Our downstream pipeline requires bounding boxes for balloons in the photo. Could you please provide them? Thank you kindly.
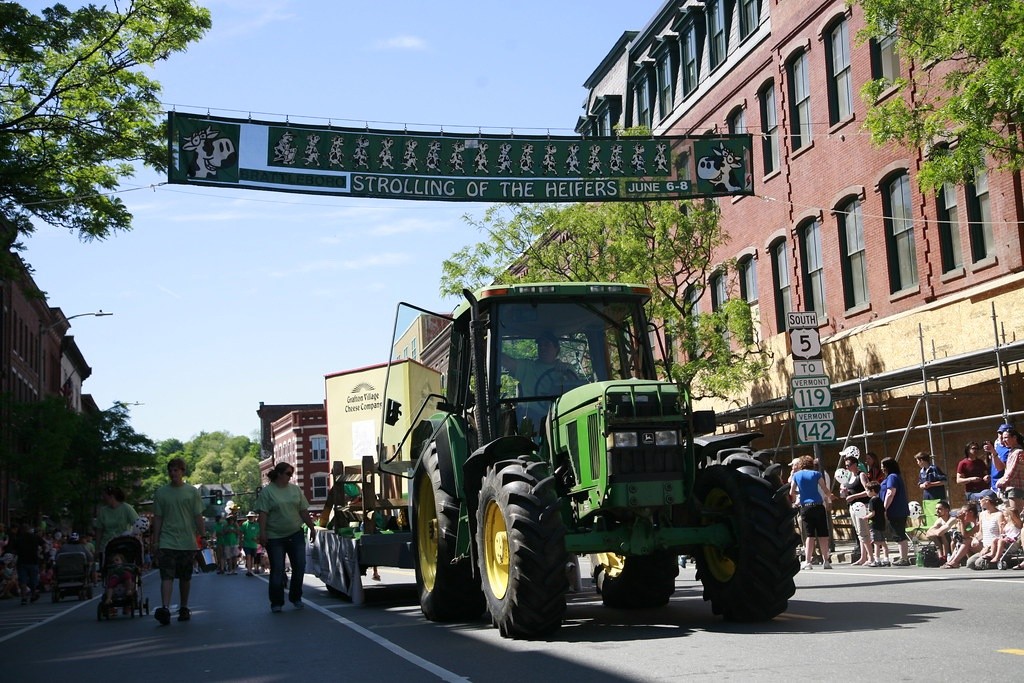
[843,446,860,461]
[850,502,866,518]
[835,468,848,484]
[907,502,922,518]
[134,516,149,533]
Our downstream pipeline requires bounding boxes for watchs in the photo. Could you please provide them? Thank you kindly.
[200,535,209,540]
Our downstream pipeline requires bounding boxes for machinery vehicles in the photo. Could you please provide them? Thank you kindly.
[301,282,803,641]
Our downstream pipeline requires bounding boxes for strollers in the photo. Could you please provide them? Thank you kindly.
[96,533,149,621]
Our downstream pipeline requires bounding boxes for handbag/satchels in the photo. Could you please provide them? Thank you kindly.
[916,546,939,567]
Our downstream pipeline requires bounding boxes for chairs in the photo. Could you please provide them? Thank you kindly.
[903,499,951,565]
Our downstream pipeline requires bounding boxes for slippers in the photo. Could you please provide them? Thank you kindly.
[940,562,960,568]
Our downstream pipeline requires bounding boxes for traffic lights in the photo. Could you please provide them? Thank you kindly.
[256,487,262,498]
[215,490,222,505]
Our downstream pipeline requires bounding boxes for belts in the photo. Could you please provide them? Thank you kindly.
[804,502,821,506]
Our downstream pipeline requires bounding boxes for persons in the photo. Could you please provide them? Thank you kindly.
[913,423,1024,570]
[348,485,397,581]
[0,521,96,610]
[839,446,910,567]
[787,456,835,570]
[193,510,270,575]
[485,332,587,419]
[94,486,150,621]
[258,463,317,613]
[302,511,322,537]
[154,459,210,625]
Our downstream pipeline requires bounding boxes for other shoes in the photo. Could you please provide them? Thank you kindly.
[127,589,133,596]
[986,553,995,558]
[853,557,909,567]
[802,563,813,570]
[823,563,832,569]
[193,564,264,576]
[158,610,171,625]
[271,602,282,612]
[104,599,113,604]
[990,557,1001,562]
[21,594,41,606]
[293,600,304,608]
[178,607,191,620]
[1012,564,1024,570]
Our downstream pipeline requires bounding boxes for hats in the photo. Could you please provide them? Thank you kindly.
[971,490,997,501]
[247,511,257,517]
[997,424,1012,432]
[788,458,801,466]
[68,532,80,541]
[225,515,235,520]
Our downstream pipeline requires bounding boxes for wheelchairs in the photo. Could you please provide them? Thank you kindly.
[49,551,93,603]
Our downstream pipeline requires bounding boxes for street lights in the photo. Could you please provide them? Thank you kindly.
[39,309,112,402]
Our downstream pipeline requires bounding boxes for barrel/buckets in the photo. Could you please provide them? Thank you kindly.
[195,548,219,574]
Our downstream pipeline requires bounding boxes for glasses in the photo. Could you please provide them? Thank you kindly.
[998,431,1003,435]
[979,497,987,500]
[283,471,292,476]
[844,462,856,468]
[970,447,979,451]
